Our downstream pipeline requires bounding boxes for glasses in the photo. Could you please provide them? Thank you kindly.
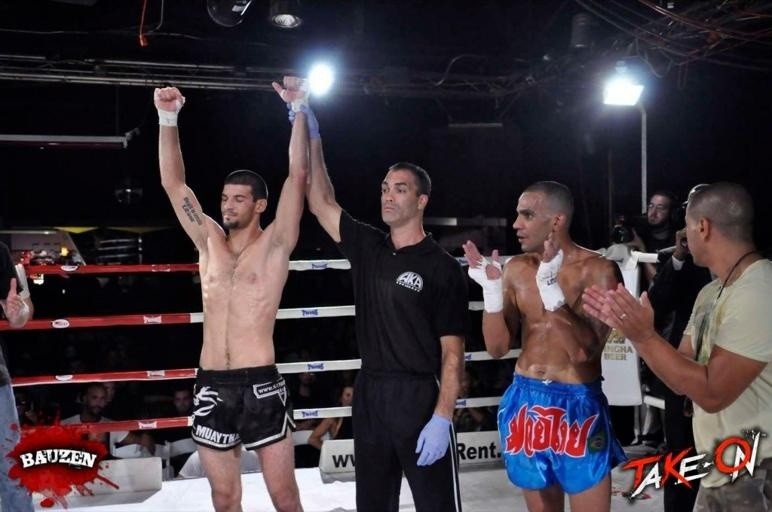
[647,203,671,211]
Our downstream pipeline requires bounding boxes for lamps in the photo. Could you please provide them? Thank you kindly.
[207,0,253,28]
[601,62,644,106]
[269,0,304,29]
[569,13,592,48]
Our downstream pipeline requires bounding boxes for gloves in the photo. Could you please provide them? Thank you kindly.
[287,104,321,140]
[415,414,451,466]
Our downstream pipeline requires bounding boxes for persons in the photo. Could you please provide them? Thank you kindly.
[460,181,628,512]
[152,76,310,512]
[287,102,468,512]
[0,242,35,512]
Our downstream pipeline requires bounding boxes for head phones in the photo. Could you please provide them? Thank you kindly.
[670,191,680,223]
